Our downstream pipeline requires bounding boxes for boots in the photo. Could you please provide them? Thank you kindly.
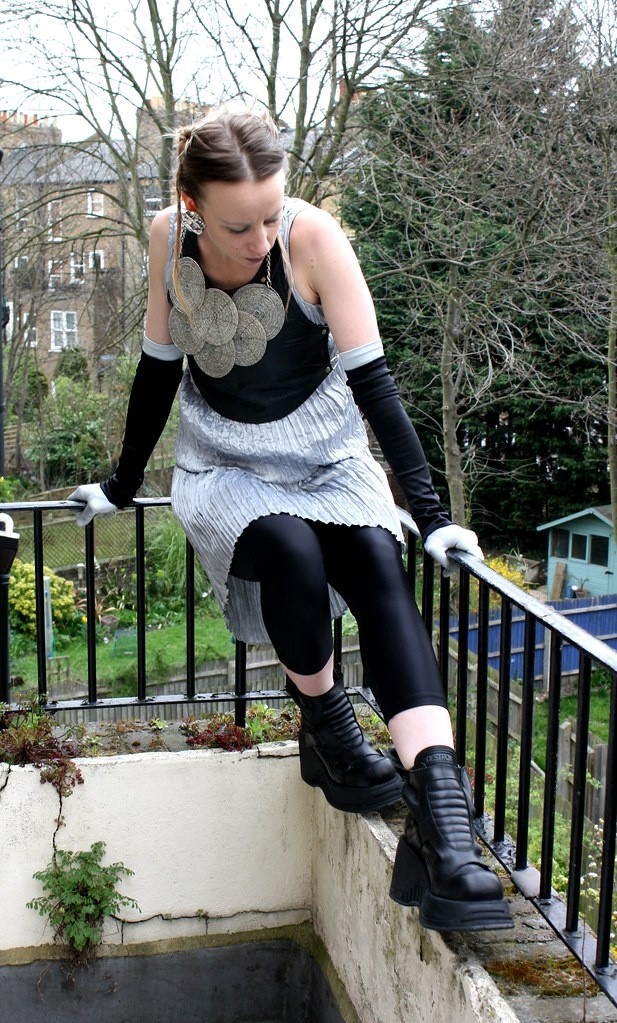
[286,668,405,813]
[388,744,515,932]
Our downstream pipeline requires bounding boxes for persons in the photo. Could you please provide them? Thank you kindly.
[70,107,514,933]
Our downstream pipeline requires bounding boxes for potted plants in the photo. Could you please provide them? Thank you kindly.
[568,574,590,598]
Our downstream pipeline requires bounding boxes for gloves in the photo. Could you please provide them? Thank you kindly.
[66,483,118,528]
[423,524,484,577]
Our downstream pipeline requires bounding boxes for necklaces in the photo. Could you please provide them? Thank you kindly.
[167,230,287,378]
